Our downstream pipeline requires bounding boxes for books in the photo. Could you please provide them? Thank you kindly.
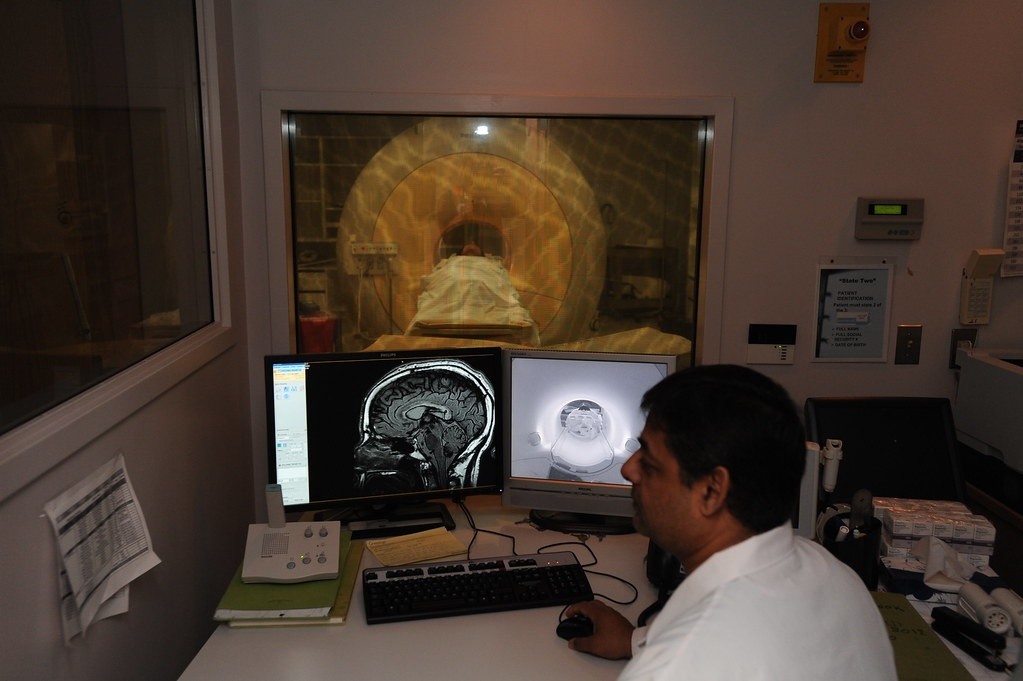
[212,530,365,628]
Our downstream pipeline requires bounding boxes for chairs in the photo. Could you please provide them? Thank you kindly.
[805,396,1001,599]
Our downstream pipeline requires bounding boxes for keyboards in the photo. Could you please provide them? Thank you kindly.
[363,550,594,624]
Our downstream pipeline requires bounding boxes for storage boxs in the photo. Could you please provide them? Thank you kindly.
[870,494,997,570]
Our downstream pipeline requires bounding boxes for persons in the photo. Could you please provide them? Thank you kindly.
[565,364,901,681]
[444,240,499,323]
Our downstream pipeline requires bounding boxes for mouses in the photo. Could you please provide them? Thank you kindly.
[556,616,595,642]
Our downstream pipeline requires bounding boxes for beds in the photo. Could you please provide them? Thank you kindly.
[410,253,535,345]
[550,428,614,473]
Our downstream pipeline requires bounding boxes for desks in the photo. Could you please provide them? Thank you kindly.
[165,504,659,681]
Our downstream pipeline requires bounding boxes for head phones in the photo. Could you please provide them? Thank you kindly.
[646,540,687,598]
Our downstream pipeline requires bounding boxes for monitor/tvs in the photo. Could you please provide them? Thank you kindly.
[505,348,677,535]
[264,347,505,539]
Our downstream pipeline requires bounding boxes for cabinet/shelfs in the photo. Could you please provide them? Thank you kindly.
[296,134,394,243]
[297,266,336,311]
